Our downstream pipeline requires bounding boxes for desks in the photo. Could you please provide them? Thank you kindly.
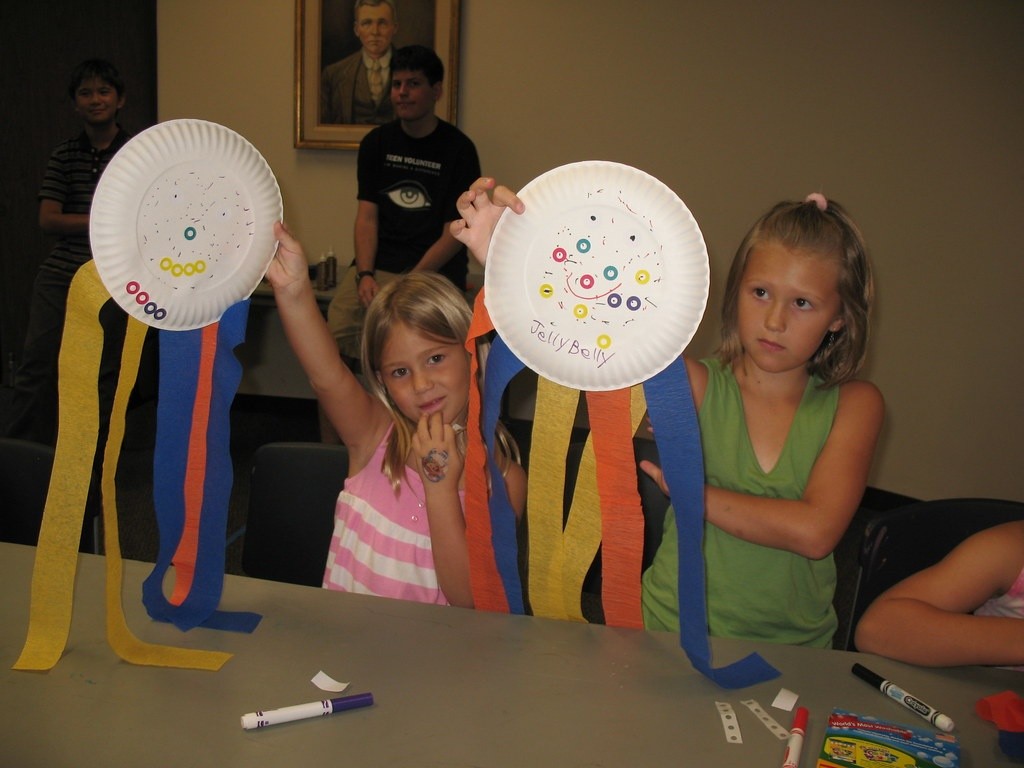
[250,259,484,318]
[0,541,1024,768]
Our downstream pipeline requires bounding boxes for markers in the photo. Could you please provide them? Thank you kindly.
[240,692,374,730]
[781,707,809,768]
[851,661,956,733]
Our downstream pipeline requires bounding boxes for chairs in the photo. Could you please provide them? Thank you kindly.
[845,496,1024,654]
[217,387,917,671]
[0,438,101,558]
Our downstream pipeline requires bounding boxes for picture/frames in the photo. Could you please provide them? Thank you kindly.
[293,0,462,151]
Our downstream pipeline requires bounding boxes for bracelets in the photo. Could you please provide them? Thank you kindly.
[353,269,376,284]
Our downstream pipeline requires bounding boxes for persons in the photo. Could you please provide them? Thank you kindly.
[266,220,527,611]
[854,518,1024,668]
[450,175,885,648]
[2,56,133,443]
[314,44,481,447]
